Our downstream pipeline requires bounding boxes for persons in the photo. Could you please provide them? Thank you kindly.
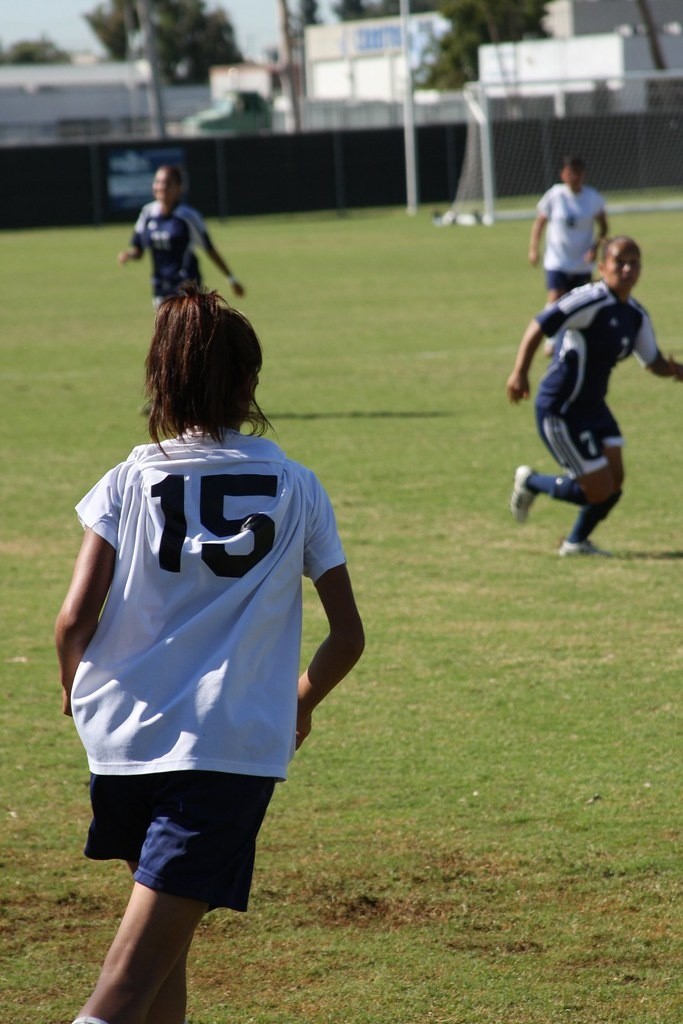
[119,163,244,314]
[508,236,683,560]
[53,284,364,1024]
[528,156,609,356]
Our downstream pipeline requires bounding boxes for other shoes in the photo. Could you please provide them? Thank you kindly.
[544,334,558,357]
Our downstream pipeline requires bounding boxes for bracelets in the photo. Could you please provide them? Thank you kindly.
[228,275,239,284]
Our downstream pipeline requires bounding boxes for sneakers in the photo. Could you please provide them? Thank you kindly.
[559,538,612,559]
[509,465,537,524]
